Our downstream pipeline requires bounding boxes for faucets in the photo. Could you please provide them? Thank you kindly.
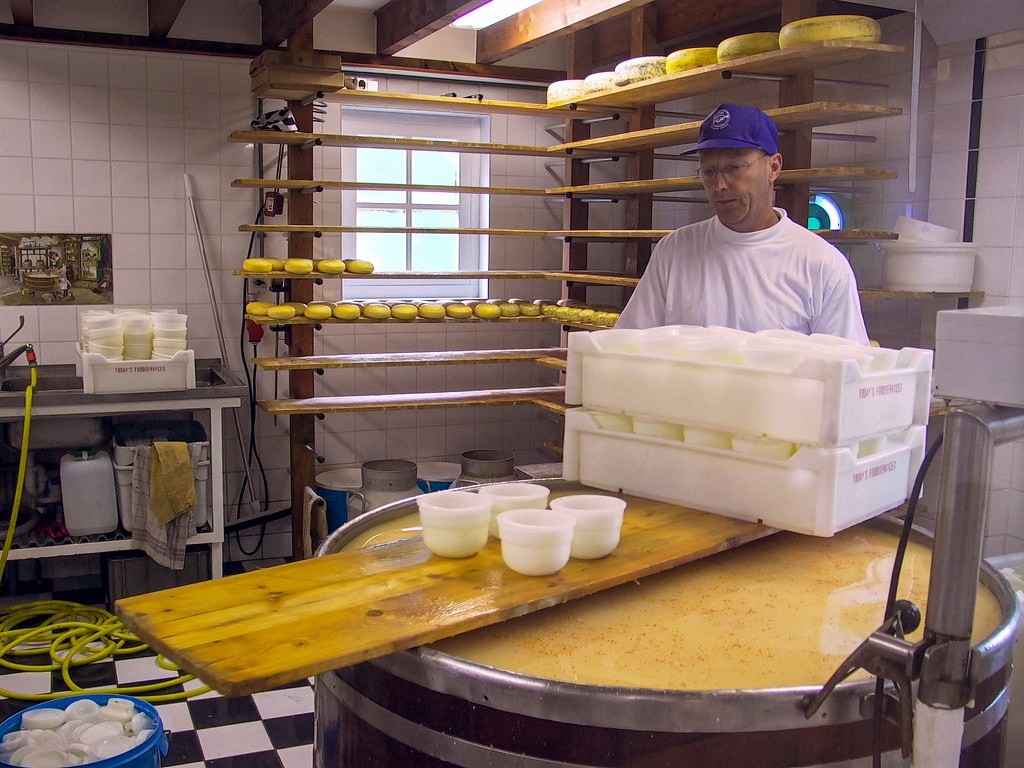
[0,314,39,381]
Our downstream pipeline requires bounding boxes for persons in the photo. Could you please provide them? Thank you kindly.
[612,103,871,348]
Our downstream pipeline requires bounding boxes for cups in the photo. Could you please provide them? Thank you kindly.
[549,495,628,560]
[496,508,578,576]
[416,491,492,558]
[478,483,551,540]
[80,308,189,362]
[589,412,900,463]
[591,323,900,378]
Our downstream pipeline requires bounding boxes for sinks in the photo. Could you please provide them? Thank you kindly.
[0,377,104,451]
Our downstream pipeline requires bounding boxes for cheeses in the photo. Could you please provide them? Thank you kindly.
[242,257,374,273]
[778,15,881,50]
[666,47,718,75]
[547,79,584,103]
[717,32,779,63]
[614,56,666,86]
[584,72,614,95]
[246,298,624,325]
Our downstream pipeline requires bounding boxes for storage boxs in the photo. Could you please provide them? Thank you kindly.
[251,68,345,101]
[75,340,196,395]
[933,305,1024,407]
[109,419,211,532]
[249,50,341,76]
[561,325,935,538]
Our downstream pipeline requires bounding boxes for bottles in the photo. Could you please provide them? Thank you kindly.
[59,449,119,537]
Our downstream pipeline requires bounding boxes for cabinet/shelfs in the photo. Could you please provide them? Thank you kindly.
[1,397,241,580]
[227,36,915,417]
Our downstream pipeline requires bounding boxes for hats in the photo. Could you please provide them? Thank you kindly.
[679,103,778,156]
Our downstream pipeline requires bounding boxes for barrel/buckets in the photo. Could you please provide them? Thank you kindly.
[315,449,518,534]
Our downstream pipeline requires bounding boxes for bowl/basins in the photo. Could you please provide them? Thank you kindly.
[876,240,979,293]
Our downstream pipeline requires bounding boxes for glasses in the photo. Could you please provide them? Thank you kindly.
[694,153,767,179]
